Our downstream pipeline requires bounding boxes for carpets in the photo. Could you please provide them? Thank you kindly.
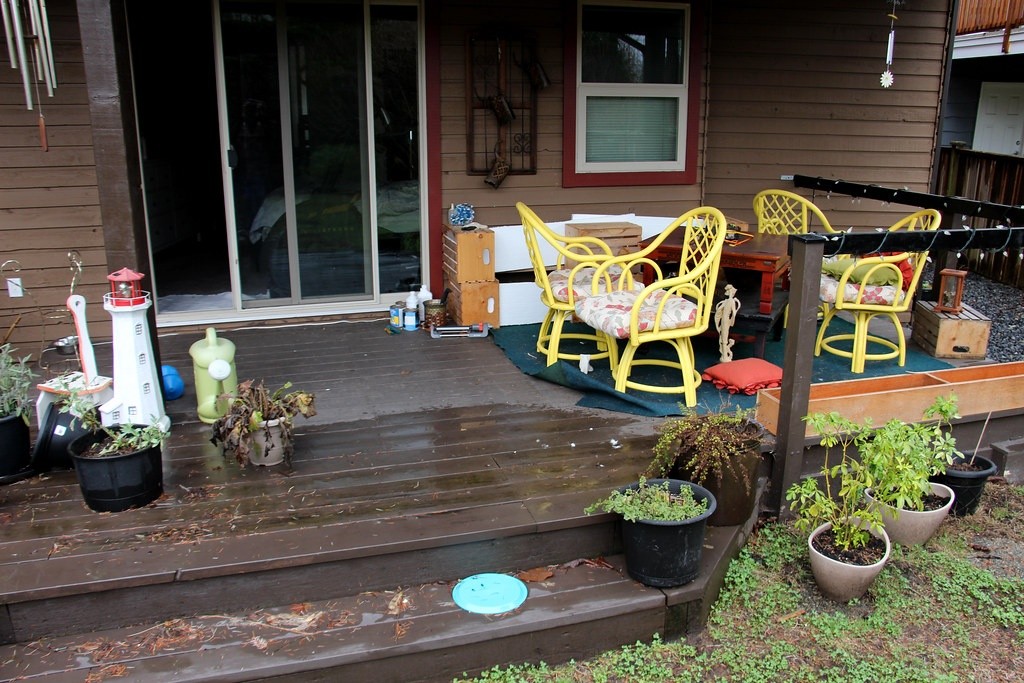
[491,316,957,417]
[158,289,270,314]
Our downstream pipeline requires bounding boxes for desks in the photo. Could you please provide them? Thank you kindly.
[638,225,790,360]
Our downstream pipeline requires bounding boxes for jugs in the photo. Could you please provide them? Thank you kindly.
[189,327,239,425]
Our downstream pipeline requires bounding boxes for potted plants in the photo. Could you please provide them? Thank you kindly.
[0,343,41,475]
[786,390,996,600]
[583,392,779,587]
[209,377,318,469]
[54,371,171,512]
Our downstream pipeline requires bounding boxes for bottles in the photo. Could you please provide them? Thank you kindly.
[417,286,432,321]
[161,364,185,400]
[406,290,419,310]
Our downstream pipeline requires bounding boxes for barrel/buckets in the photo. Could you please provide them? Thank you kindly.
[389,301,421,331]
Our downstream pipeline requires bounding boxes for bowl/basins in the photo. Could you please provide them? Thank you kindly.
[53,336,79,355]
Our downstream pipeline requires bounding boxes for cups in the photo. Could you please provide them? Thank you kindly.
[422,299,448,327]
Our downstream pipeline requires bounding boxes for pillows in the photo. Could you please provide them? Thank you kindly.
[821,258,899,287]
[702,357,783,395]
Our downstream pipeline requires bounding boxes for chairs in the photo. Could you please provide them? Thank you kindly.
[753,189,941,373]
[515,202,727,409]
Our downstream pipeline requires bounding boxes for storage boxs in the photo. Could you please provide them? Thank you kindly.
[755,363,1024,437]
[566,223,644,323]
[932,267,968,315]
[911,300,992,361]
[442,223,501,330]
